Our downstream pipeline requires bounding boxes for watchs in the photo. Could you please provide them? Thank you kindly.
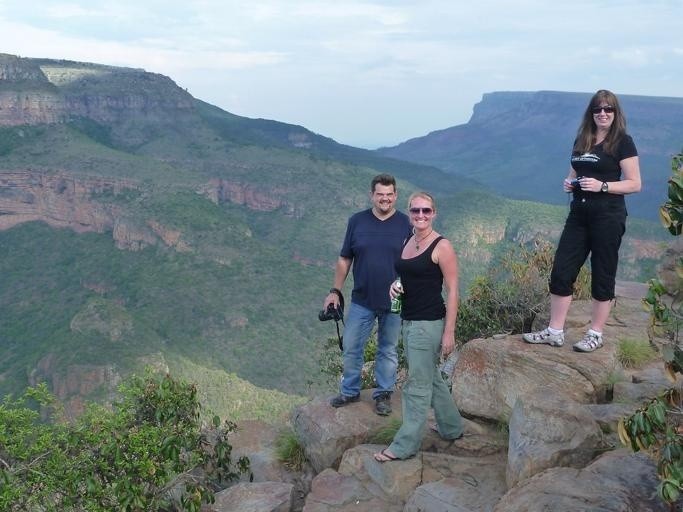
[601,179,609,195]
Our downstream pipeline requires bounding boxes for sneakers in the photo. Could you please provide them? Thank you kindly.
[375,393,392,415]
[573,332,603,352]
[330,393,360,406]
[523,329,564,346]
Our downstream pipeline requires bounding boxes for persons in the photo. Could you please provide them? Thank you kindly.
[373,190,458,464]
[323,172,411,415]
[521,89,643,352]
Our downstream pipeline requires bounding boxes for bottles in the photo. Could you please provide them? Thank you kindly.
[390,277,402,315]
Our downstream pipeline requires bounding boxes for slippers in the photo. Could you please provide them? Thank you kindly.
[375,448,402,463]
[431,424,464,438]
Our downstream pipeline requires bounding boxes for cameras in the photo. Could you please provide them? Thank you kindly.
[571,180,581,193]
[319,304,345,321]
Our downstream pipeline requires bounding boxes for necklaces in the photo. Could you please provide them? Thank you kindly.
[413,229,434,252]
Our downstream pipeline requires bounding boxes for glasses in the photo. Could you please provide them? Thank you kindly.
[591,105,616,113]
[410,208,432,216]
